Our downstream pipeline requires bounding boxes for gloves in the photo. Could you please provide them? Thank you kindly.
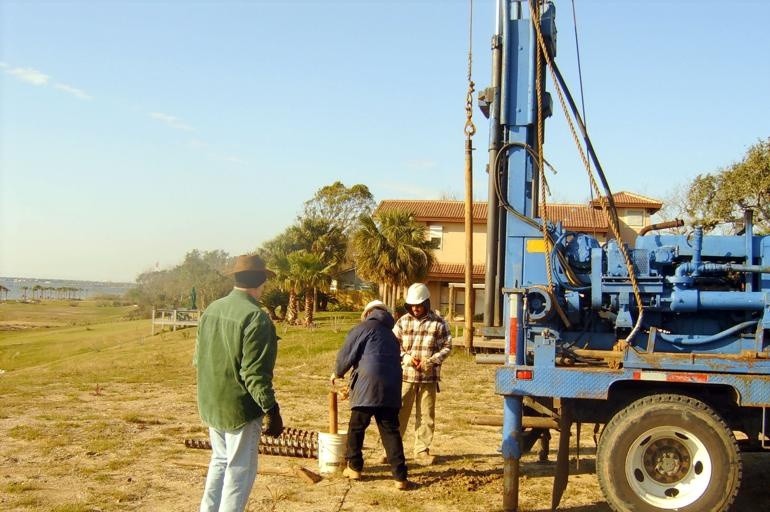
[261,404,283,438]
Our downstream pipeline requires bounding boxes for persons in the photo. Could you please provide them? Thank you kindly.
[392,282,452,466]
[196,254,284,512]
[330,300,409,490]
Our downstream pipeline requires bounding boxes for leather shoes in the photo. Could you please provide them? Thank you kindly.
[413,452,436,462]
[394,479,408,489]
[343,468,361,479]
[378,455,387,464]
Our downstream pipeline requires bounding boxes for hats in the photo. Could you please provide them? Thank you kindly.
[224,254,276,278]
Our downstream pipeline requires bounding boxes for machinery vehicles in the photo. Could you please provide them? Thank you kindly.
[460,1,769,512]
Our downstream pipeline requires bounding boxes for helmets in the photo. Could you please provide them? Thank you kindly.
[360,299,387,321]
[405,282,431,305]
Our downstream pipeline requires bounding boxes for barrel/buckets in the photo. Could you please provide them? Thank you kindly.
[318,429,349,478]
